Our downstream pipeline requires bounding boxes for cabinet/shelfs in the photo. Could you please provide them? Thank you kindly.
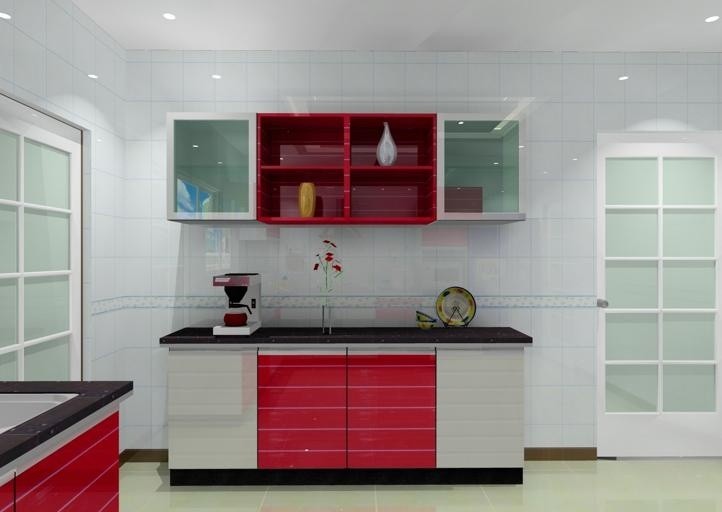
[256,114,438,223]
[437,115,526,221]
[168,349,258,470]
[437,351,524,471]
[1,410,121,512]
[166,114,257,221]
[257,347,435,470]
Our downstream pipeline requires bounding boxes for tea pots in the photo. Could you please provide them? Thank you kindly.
[223,304,252,327]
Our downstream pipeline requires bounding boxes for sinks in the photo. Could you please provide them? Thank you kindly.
[0,388,84,433]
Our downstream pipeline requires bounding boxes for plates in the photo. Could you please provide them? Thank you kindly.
[436,286,476,326]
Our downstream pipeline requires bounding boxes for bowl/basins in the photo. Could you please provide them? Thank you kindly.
[415,311,437,331]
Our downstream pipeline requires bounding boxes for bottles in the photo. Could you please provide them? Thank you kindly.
[298,182,316,218]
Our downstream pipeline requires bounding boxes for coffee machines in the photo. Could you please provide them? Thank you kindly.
[212,272,263,335]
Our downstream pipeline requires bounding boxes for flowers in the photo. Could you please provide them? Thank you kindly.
[312,239,346,299]
